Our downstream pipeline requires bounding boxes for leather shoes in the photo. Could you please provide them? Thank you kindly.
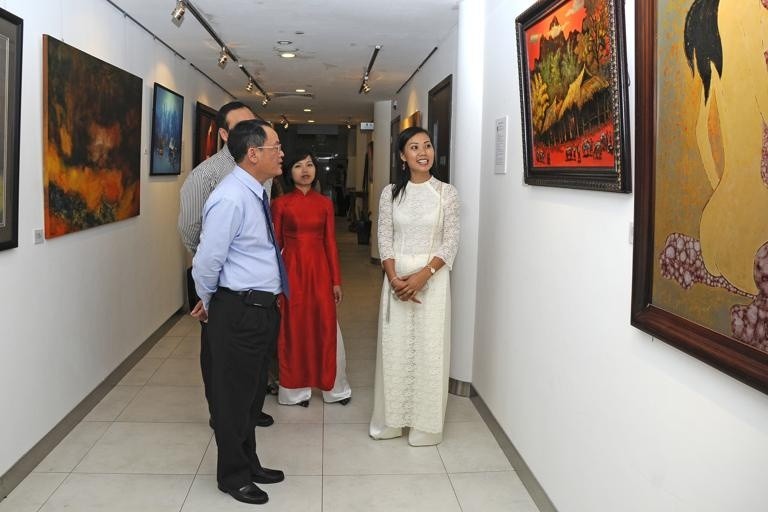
[256,413,274,426]
[218,482,269,504]
[255,467,285,484]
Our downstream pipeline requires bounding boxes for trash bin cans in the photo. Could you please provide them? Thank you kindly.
[355,221,371,244]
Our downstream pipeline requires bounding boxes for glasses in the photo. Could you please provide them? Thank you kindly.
[258,143,282,152]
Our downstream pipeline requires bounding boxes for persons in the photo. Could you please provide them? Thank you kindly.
[368,125,461,447]
[269,149,353,409]
[190,119,291,504]
[681,2,768,295]
[178,100,274,430]
[333,163,348,217]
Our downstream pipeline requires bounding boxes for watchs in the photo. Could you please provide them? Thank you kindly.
[425,263,436,275]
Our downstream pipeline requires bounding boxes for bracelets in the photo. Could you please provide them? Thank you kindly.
[389,276,399,287]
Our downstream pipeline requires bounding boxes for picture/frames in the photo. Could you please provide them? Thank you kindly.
[192,102,219,171]
[515,0,632,194]
[389,115,401,184]
[149,83,184,176]
[630,1,767,396]
[0,8,23,251]
[427,73,451,185]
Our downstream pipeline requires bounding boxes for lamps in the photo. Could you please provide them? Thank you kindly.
[171,1,269,109]
[277,113,291,129]
[361,72,371,96]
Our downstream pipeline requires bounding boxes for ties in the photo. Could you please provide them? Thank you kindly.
[263,191,289,299]
